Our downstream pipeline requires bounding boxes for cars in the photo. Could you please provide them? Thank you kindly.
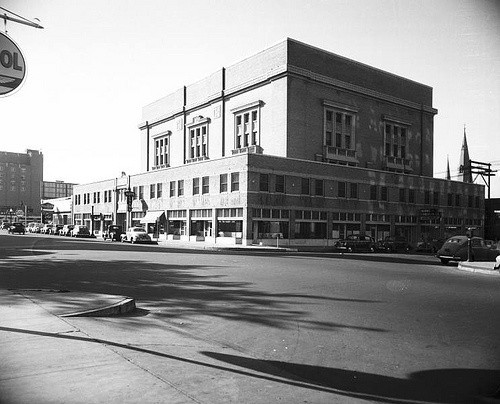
[437,236,500,265]
[2,221,151,243]
[338,234,378,253]
[377,234,416,253]
